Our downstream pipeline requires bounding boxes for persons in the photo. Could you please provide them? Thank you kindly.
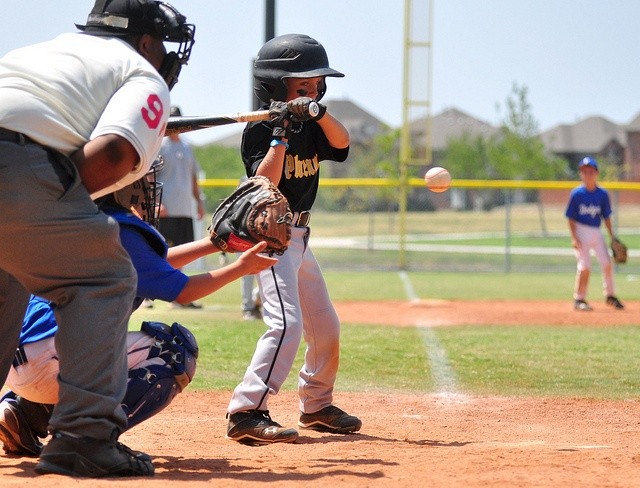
[566,157,627,313]
[142,105,206,308]
[0,0,195,477]
[224,34,361,443]
[0,152,278,460]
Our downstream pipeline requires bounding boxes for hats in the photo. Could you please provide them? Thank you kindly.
[579,158,597,168]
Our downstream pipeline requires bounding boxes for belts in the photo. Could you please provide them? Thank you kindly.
[0,127,39,145]
[290,211,311,227]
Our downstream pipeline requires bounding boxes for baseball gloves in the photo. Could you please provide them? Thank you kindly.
[210,172,294,256]
[611,238,629,264]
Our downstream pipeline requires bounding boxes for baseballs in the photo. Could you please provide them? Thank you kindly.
[426,166,452,192]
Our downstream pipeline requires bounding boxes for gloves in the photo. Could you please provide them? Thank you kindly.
[286,97,327,121]
[269,102,289,140]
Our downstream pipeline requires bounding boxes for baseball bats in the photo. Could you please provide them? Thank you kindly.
[162,100,320,136]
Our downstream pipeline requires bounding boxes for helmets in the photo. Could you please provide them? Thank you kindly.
[96,153,163,227]
[74,0,195,90]
[253,35,344,105]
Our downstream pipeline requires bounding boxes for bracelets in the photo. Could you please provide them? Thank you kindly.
[270,138,290,147]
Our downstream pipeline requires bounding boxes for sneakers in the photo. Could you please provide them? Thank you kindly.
[242,306,263,322]
[0,398,45,454]
[575,301,593,311]
[35,432,153,477]
[226,409,299,447]
[606,297,624,310]
[299,405,362,432]
